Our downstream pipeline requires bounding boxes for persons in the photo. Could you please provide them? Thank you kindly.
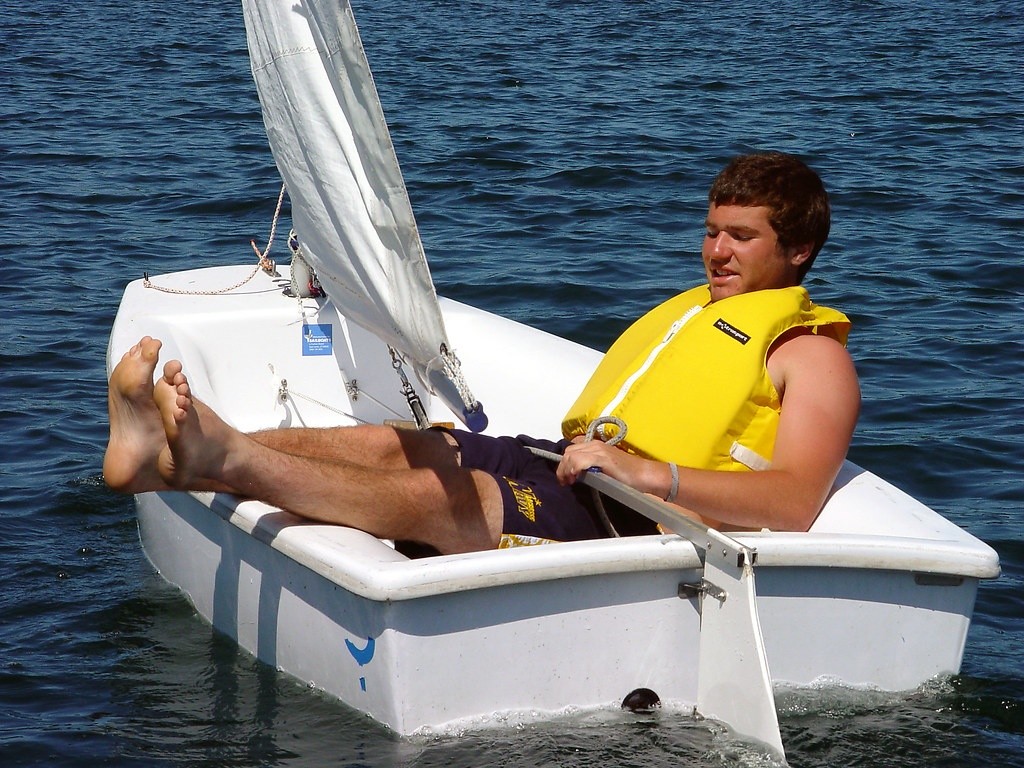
[101,154,861,560]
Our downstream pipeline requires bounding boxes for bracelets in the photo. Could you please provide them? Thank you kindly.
[663,463,679,504]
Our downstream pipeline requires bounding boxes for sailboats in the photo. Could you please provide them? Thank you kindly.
[101,1,1001,739]
